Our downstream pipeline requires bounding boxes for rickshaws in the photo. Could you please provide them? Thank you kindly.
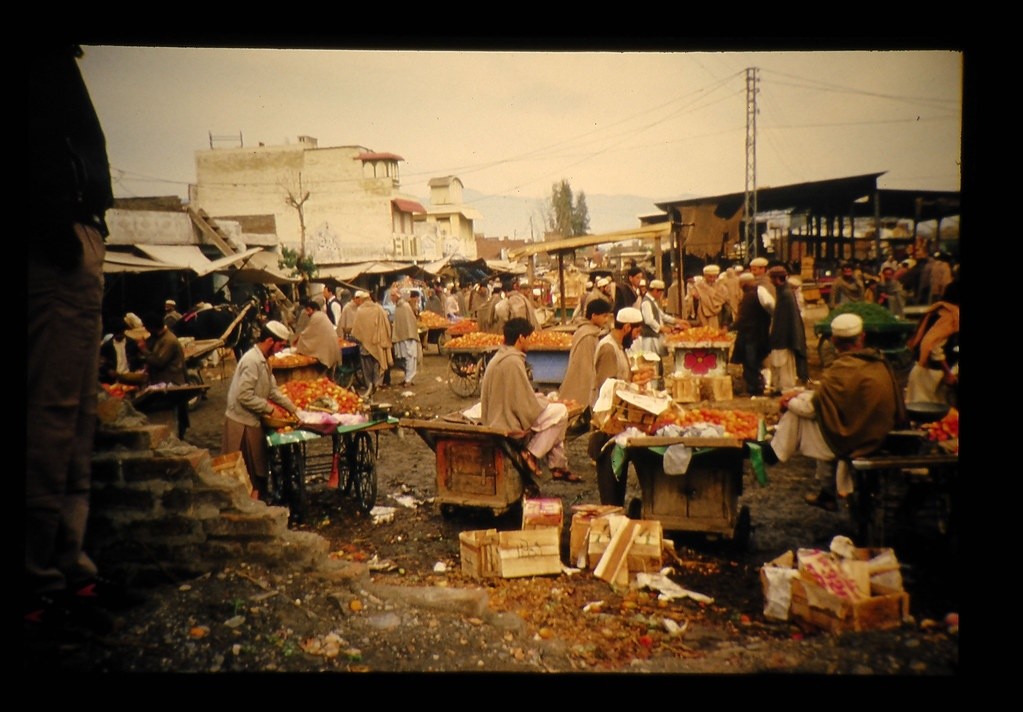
[123,345,958,549]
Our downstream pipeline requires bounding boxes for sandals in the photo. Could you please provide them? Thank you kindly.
[519,449,543,480]
[551,467,586,483]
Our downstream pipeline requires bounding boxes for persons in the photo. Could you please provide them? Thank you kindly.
[91,258,961,511]
[10,45,111,591]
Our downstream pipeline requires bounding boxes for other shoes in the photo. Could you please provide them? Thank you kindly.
[804,488,838,511]
[745,440,778,466]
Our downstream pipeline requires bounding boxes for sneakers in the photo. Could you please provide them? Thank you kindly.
[22,589,126,635]
[61,568,150,611]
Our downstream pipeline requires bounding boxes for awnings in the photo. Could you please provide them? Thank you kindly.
[101,246,528,291]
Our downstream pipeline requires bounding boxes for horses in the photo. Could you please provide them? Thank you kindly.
[170,282,277,362]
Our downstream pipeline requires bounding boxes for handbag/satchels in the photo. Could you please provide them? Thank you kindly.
[588,431,610,462]
[756,284,775,315]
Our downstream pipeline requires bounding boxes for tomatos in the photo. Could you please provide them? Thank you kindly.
[276,376,364,433]
[103,382,134,399]
[675,408,758,440]
[925,420,959,442]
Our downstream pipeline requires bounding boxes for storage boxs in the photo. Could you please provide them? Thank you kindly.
[761,544,911,646]
[206,451,254,494]
[458,496,664,583]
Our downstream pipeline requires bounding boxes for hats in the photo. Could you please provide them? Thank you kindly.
[649,280,666,289]
[616,307,643,323]
[831,313,863,337]
[265,320,290,340]
[165,300,176,306]
[750,256,768,268]
[703,264,720,275]
[354,289,370,299]
[735,265,743,272]
[585,274,612,289]
[770,266,787,277]
